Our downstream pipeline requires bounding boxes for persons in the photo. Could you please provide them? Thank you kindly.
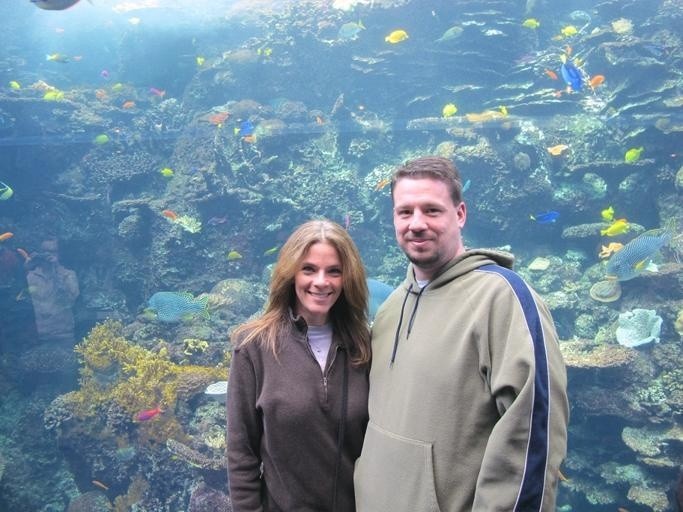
[351,155,571,512]
[224,218,372,512]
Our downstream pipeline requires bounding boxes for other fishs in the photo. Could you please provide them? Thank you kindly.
[434,0,683,283]
[1,0,279,263]
